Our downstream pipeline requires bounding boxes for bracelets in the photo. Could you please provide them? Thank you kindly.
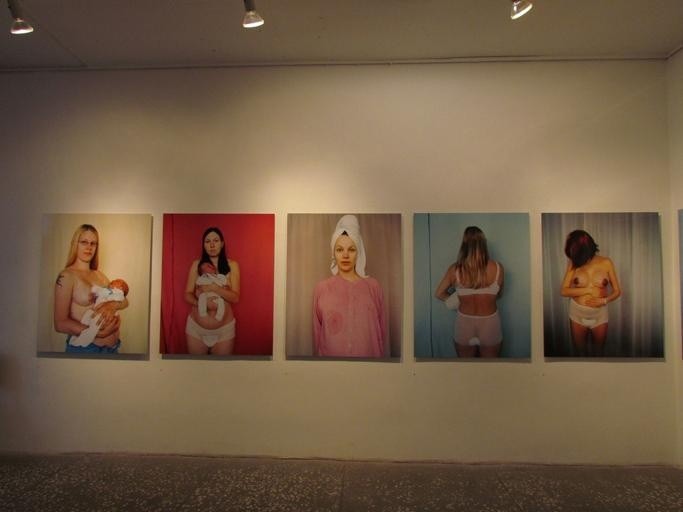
[605,299,608,306]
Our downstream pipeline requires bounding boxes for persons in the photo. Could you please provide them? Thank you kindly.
[183,227,241,356]
[435,226,504,358]
[53,224,129,354]
[197,260,231,323]
[311,214,386,357]
[81,279,128,338]
[559,229,621,356]
